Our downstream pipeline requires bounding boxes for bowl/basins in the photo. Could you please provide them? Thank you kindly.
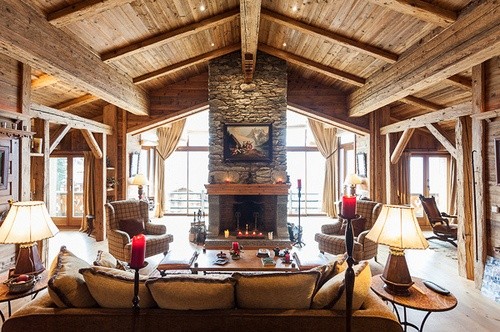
[7,274,35,293]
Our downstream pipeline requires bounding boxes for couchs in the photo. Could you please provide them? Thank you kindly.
[0,290,404,332]
[105,199,173,265]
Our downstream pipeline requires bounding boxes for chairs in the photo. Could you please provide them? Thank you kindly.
[419,194,458,247]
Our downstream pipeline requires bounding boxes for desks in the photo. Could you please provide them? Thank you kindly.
[371,274,457,332]
[189,249,299,276]
[0,267,51,323]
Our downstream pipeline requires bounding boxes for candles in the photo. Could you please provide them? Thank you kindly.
[342,193,359,218]
[298,179,302,188]
[132,234,145,266]
[232,242,240,253]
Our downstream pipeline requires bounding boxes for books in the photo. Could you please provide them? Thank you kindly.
[257,248,270,258]
[213,257,228,265]
[261,257,276,266]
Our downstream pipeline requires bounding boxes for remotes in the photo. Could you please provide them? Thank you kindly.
[422,279,450,295]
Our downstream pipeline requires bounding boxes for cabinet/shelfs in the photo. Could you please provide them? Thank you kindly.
[105,167,117,203]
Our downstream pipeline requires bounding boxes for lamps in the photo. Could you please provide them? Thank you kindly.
[365,203,428,294]
[346,174,363,198]
[132,174,148,201]
[0,201,59,276]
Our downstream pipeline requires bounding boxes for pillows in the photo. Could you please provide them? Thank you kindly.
[117,218,146,239]
[47,243,373,309]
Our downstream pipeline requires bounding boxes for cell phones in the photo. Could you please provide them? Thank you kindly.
[214,258,228,265]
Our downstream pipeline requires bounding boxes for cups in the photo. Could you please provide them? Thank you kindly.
[268,231,273,240]
[224,230,229,238]
[33,138,43,153]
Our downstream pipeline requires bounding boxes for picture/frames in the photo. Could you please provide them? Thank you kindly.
[223,123,273,164]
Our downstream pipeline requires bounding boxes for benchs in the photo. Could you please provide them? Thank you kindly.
[293,250,325,272]
[157,247,196,275]
